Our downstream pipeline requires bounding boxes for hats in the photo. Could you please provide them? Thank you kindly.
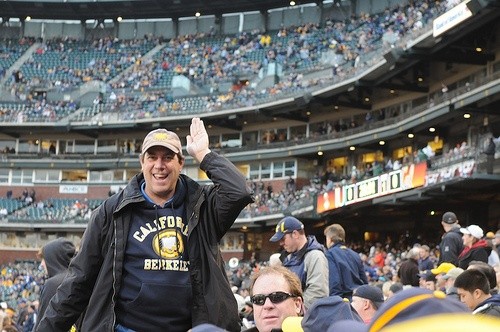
[460,225,484,239]
[353,285,383,309]
[140,129,182,157]
[430,263,456,274]
[442,212,457,224]
[443,268,463,279]
[269,216,304,242]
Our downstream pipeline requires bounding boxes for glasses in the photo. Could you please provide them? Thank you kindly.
[251,291,298,305]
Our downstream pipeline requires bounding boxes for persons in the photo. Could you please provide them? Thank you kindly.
[35,116,256,332]
[1,0,500,332]
